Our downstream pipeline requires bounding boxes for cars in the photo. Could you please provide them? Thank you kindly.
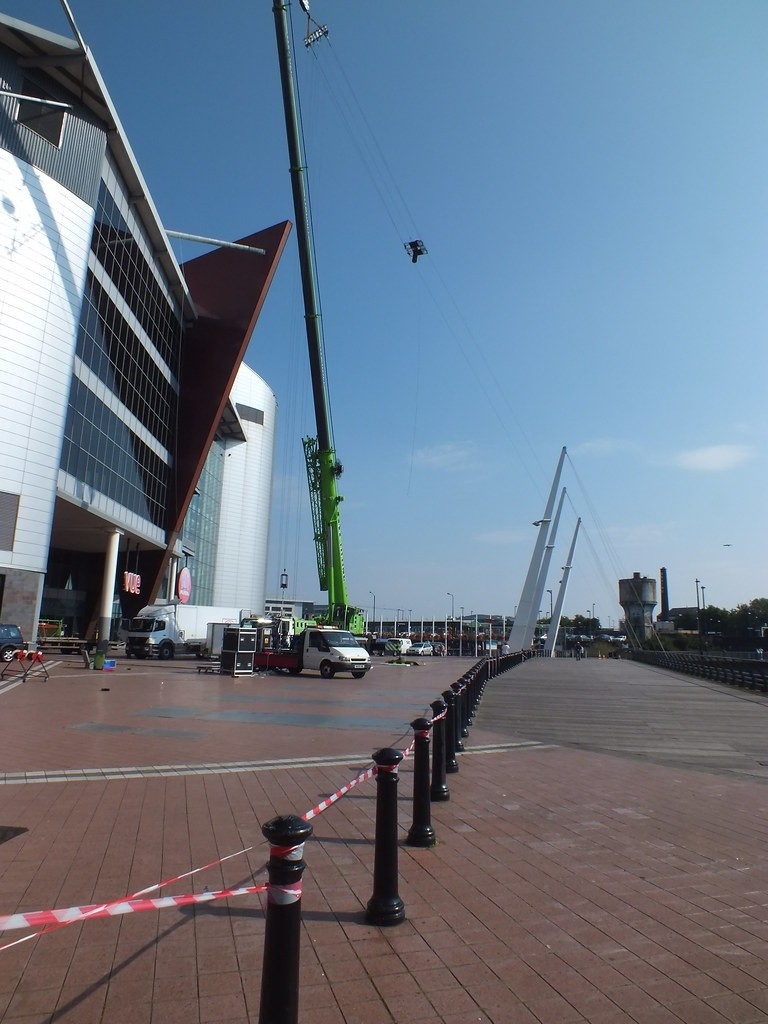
[344,637,446,657]
[531,632,627,645]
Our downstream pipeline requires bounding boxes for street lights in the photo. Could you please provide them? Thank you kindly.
[695,578,701,633]
[368,590,597,657]
[700,585,706,610]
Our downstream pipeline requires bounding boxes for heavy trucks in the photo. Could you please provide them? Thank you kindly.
[125,602,261,661]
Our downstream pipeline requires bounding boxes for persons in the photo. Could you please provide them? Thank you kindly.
[477,644,481,657]
[755,647,764,660]
[440,643,445,656]
[575,642,582,661]
[502,642,511,655]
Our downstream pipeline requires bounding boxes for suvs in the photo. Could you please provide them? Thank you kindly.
[0,623,24,662]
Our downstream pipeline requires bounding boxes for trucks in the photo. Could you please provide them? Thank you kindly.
[256,623,373,678]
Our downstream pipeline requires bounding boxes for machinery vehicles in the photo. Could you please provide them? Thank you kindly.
[267,0,379,655]
